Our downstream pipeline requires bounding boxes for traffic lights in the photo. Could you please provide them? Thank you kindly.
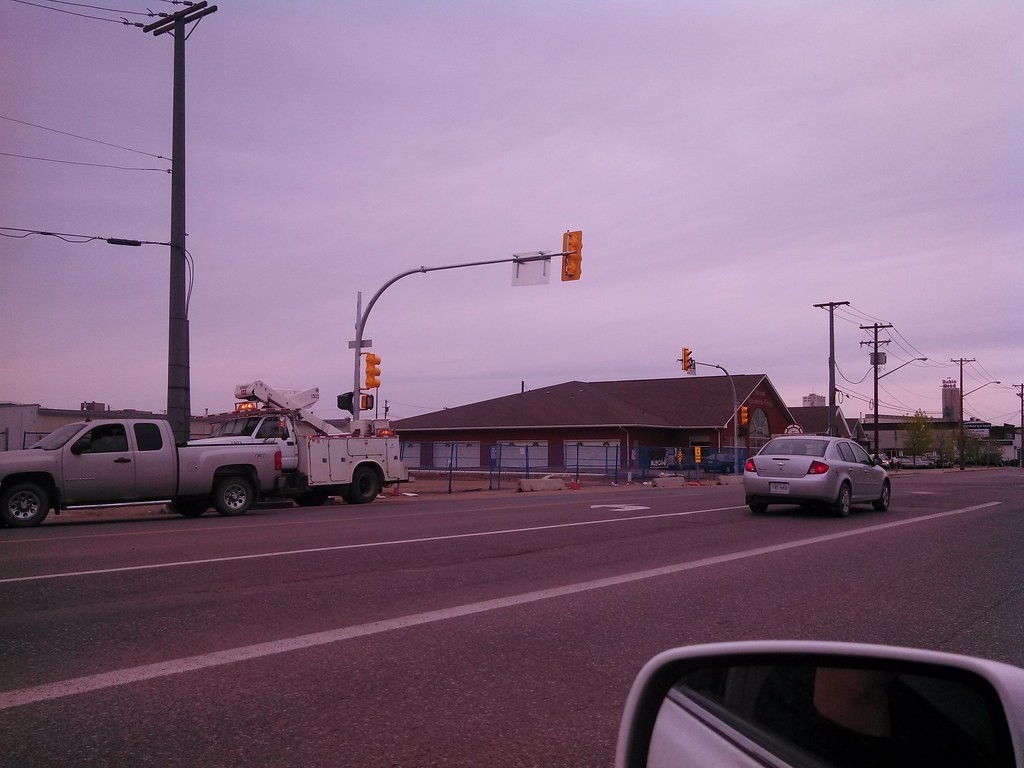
[561,230,582,280]
[741,406,749,426]
[682,347,692,370]
[366,353,381,387]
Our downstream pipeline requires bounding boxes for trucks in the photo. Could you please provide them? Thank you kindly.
[187,378,410,504]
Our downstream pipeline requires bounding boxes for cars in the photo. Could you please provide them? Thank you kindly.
[702,453,745,474]
[869,451,953,469]
[742,435,892,519]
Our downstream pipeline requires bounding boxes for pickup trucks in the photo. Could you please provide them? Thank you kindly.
[0,419,282,530]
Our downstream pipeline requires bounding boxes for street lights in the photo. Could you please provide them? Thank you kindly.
[859,322,927,461]
[951,358,1001,470]
